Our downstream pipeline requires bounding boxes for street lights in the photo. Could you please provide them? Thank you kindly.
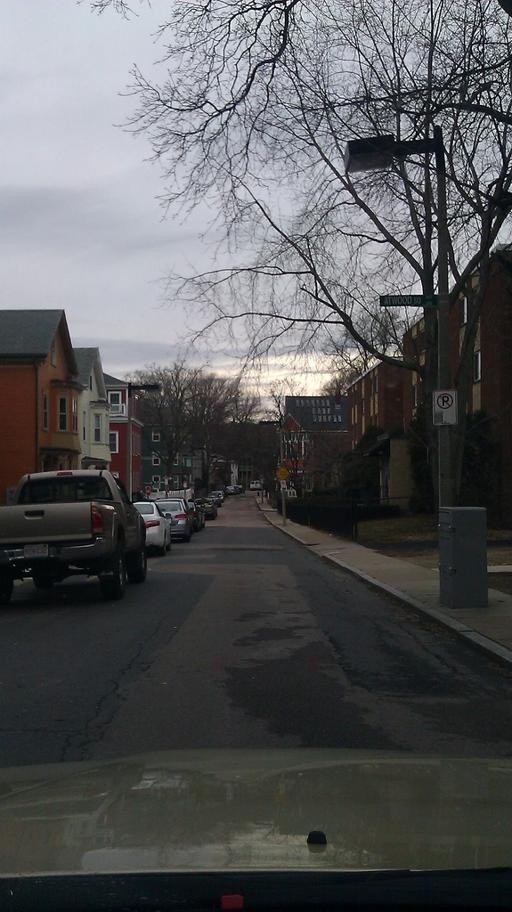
[344,124,452,507]
[259,420,287,525]
[127,382,159,502]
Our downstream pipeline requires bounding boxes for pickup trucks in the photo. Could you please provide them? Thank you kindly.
[0,470,146,608]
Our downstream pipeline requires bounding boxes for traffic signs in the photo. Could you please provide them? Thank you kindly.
[379,295,423,306]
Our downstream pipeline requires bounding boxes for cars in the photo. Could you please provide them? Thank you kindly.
[226,485,243,495]
[133,501,171,556]
[155,490,227,542]
